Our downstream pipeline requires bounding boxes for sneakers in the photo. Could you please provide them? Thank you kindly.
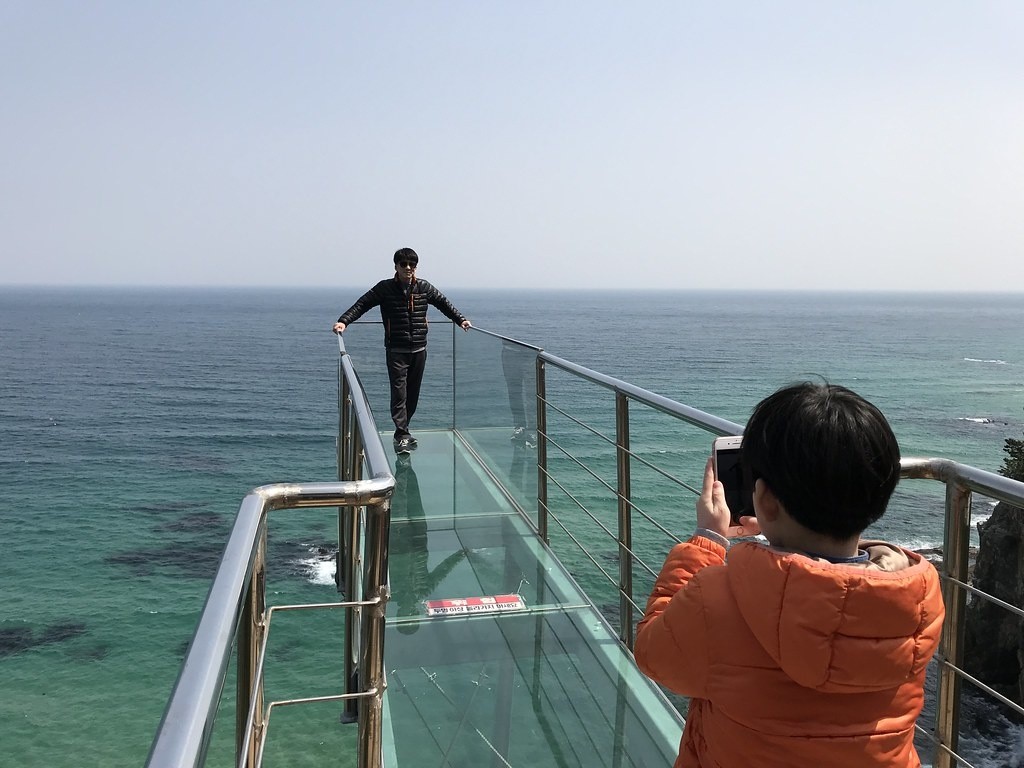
[410,437,418,445]
[395,438,411,455]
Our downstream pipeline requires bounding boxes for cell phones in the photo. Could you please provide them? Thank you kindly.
[712,436,762,538]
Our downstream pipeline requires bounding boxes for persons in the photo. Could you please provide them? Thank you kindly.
[332,247,472,455]
[633,372,947,768]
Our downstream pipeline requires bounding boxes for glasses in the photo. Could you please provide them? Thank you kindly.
[397,261,417,268]
